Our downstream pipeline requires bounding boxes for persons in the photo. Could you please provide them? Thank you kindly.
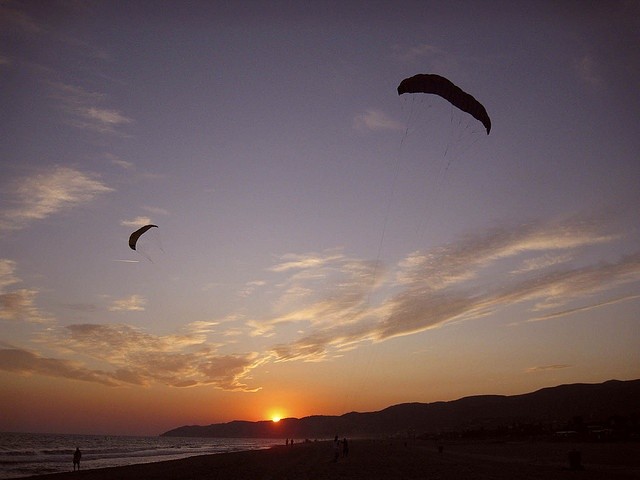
[333,435,340,462]
[285,438,289,447]
[303,439,316,445]
[291,439,294,446]
[342,438,349,458]
[73,446,81,471]
